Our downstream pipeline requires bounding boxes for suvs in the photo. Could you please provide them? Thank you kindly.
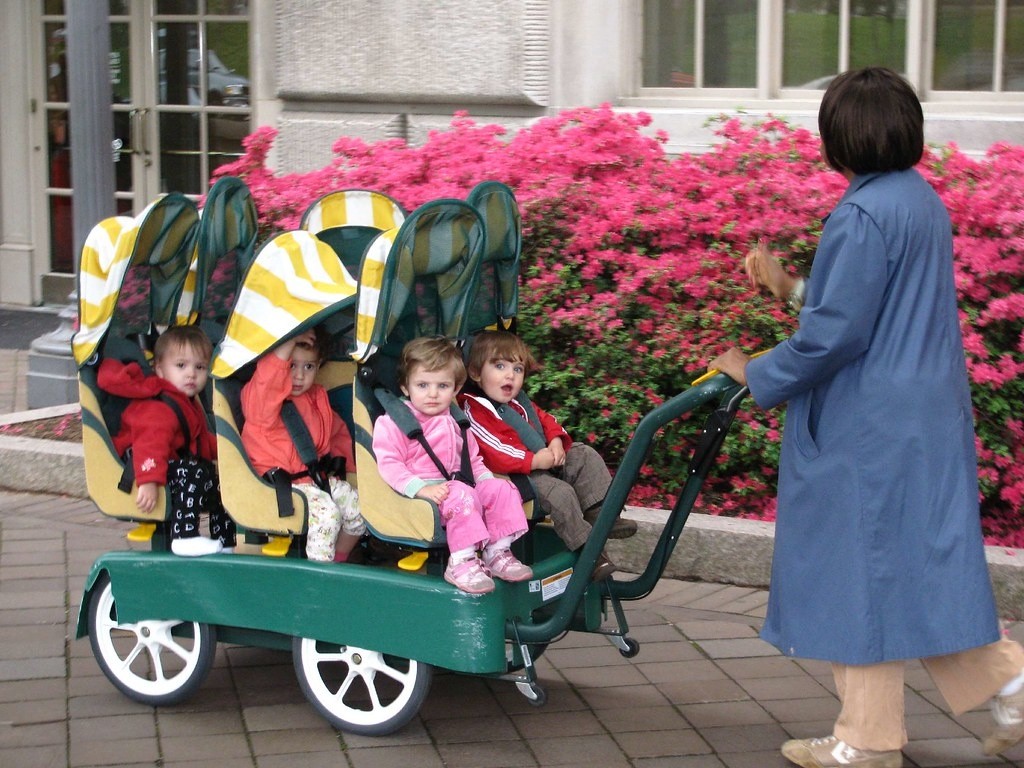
[155,48,248,113]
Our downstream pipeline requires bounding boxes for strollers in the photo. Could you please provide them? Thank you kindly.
[74,174,779,738]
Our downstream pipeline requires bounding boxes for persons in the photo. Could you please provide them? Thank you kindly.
[239,321,368,564]
[313,303,415,565]
[371,334,534,592]
[705,66,1024,768]
[97,324,238,555]
[455,329,638,580]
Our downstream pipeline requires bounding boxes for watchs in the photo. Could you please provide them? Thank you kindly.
[785,279,806,312]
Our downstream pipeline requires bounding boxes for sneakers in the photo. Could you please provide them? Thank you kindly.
[591,550,617,581]
[583,506,638,539]
[782,735,903,768]
[482,548,533,581]
[982,685,1024,756]
[444,553,495,593]
